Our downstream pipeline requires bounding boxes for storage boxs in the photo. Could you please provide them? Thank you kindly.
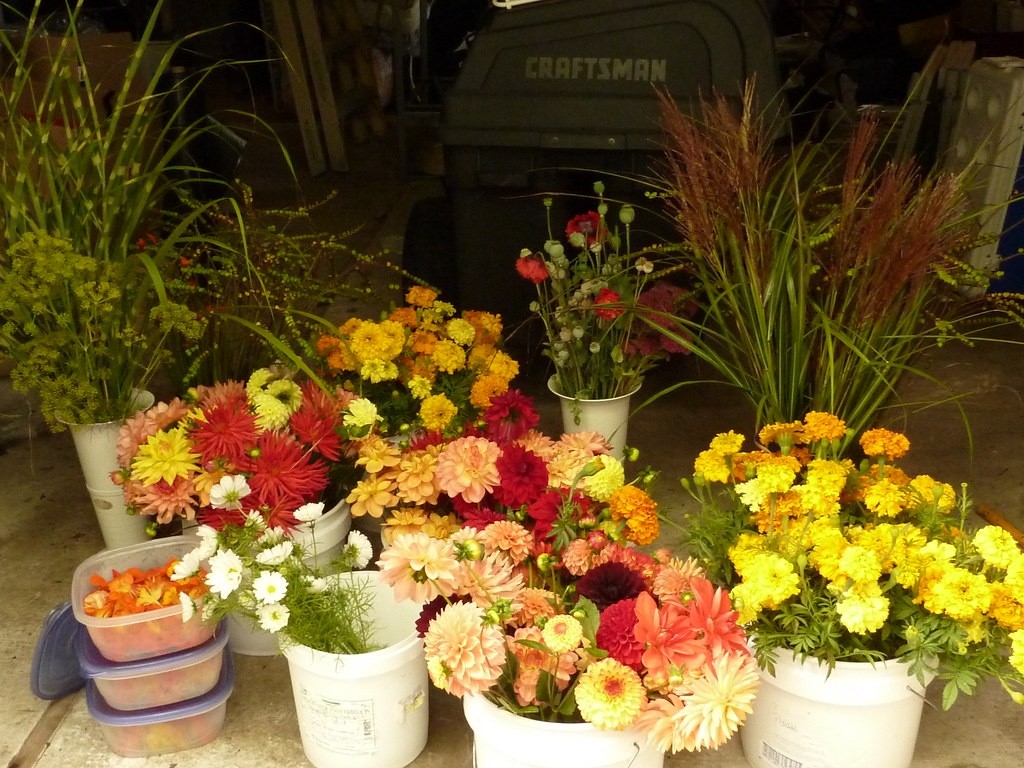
[72,534,221,662]
[66,615,229,712]
[85,642,237,758]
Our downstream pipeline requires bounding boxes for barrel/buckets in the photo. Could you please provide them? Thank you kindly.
[196,499,351,657]
[738,635,938,768]
[463,686,666,768]
[279,570,430,768]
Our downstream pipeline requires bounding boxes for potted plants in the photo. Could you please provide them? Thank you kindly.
[0,0,442,551]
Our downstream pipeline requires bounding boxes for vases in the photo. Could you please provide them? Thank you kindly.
[739,634,940,768]
[463,691,664,768]
[547,373,642,464]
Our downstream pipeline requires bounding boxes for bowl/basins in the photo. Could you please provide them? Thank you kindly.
[72,535,235,757]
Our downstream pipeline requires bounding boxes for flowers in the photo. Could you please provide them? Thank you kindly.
[345,389,762,754]
[169,475,390,662]
[515,180,701,427]
[103,359,343,542]
[313,283,521,443]
[658,407,1024,712]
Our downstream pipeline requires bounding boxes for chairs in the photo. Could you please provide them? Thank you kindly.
[824,44,948,164]
